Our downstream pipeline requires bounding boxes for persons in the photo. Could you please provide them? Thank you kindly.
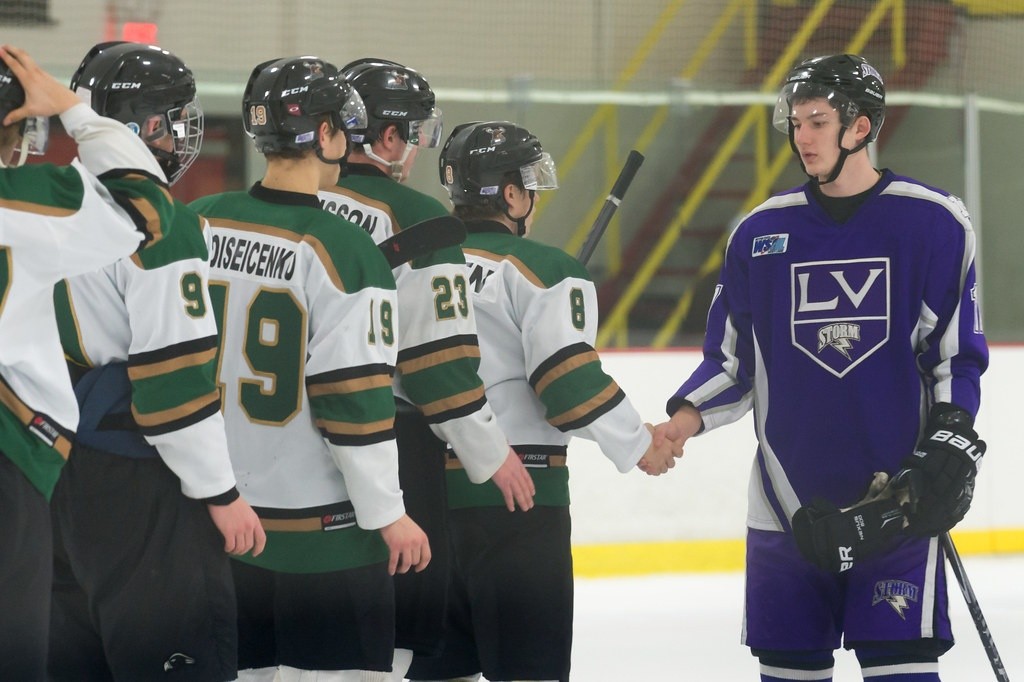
[1,40,679,682]
[639,53,989,682]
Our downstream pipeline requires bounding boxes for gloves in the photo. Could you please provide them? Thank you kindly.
[791,468,918,576]
[894,402,987,538]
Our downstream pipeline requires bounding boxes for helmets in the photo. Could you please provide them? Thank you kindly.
[438,120,542,206]
[0,53,37,136]
[338,57,435,147]
[70,41,204,188]
[785,53,885,142]
[242,56,355,153]
